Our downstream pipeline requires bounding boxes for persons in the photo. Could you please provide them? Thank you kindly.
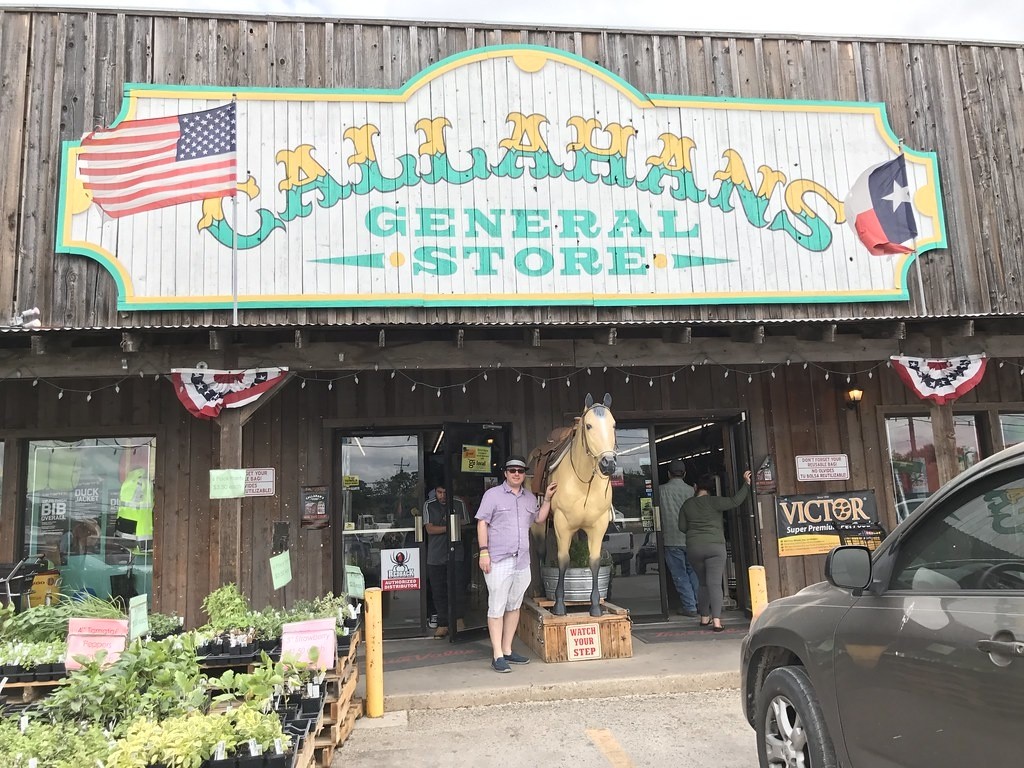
[381,532,406,549]
[659,459,700,618]
[678,469,752,632]
[423,476,471,640]
[474,454,559,674]
[60,527,74,554]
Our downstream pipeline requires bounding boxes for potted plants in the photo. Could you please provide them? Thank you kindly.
[540,539,614,601]
[0,581,362,768]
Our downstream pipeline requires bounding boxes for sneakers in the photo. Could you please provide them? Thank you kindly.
[428,613,438,629]
[434,627,449,640]
[455,618,464,632]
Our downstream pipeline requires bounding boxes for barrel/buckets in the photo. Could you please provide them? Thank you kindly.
[24,570,61,608]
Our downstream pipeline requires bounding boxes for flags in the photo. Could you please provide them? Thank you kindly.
[77,102,238,221]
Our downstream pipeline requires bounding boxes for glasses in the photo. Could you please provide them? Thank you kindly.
[506,469,525,473]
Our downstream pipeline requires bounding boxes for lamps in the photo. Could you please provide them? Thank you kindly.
[844,383,863,408]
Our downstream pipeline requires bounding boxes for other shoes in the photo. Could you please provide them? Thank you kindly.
[504,651,530,664]
[675,606,703,617]
[490,657,511,672]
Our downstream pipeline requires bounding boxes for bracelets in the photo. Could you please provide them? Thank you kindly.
[478,546,489,552]
[479,553,489,557]
[543,499,551,502]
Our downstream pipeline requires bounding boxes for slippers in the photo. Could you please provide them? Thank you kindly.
[700,618,712,626]
[714,624,725,632]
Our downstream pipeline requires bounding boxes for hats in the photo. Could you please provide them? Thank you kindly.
[668,460,685,476]
[499,455,530,473]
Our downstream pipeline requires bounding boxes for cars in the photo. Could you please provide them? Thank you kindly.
[740,440,1024,768]
